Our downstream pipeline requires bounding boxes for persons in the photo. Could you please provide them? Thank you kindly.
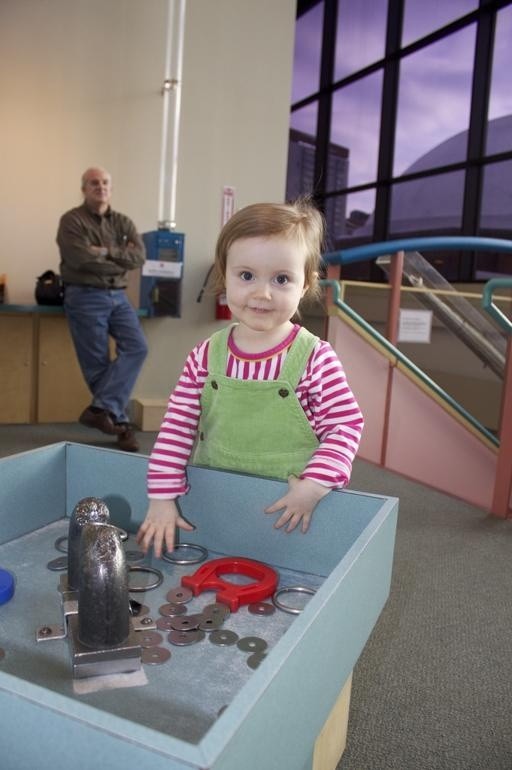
[57,166,149,451]
[136,197,366,558]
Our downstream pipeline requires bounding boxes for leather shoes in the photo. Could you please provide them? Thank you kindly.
[118,432,141,451]
[78,408,128,435]
